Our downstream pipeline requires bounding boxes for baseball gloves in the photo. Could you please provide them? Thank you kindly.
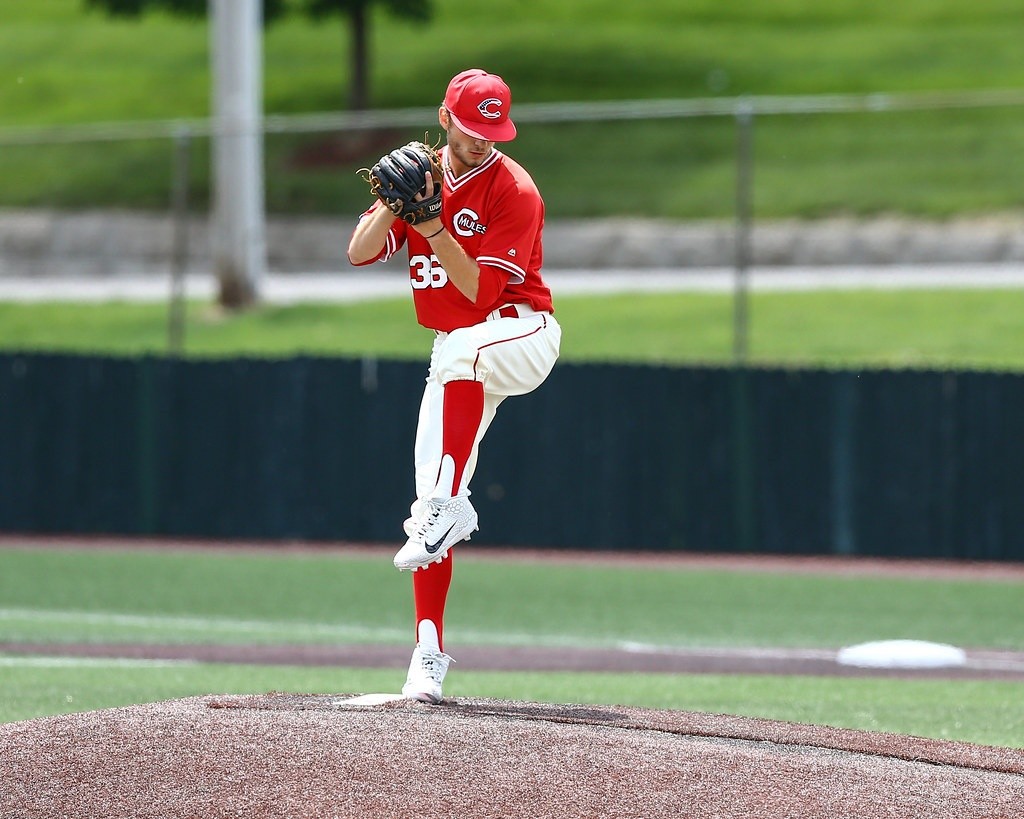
[368,140,445,224]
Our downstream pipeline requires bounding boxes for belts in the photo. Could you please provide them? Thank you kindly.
[435,304,519,336]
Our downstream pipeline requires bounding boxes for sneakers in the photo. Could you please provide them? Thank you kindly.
[394,488,480,572]
[402,643,457,705]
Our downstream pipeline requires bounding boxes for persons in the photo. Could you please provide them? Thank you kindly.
[348,69,562,704]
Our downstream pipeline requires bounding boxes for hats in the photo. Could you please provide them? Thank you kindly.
[444,68,517,142]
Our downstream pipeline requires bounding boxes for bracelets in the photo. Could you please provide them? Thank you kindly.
[425,226,444,241]
